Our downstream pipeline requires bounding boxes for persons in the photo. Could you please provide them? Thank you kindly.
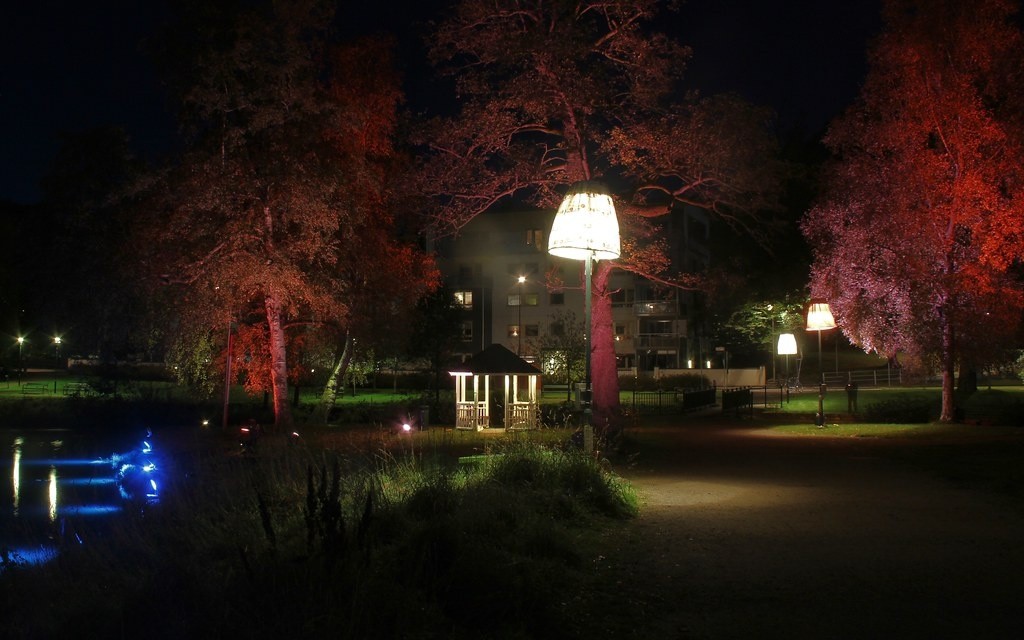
[845,374,860,415]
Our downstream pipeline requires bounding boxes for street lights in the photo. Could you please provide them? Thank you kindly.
[541,180,624,452]
[16,336,25,385]
[776,331,797,405]
[805,301,837,426]
[53,335,61,394]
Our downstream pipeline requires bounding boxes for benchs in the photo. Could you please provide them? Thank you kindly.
[22,382,49,394]
[62,382,90,395]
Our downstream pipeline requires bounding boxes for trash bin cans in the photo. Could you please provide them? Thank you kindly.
[418,404,430,431]
[582,408,592,428]
